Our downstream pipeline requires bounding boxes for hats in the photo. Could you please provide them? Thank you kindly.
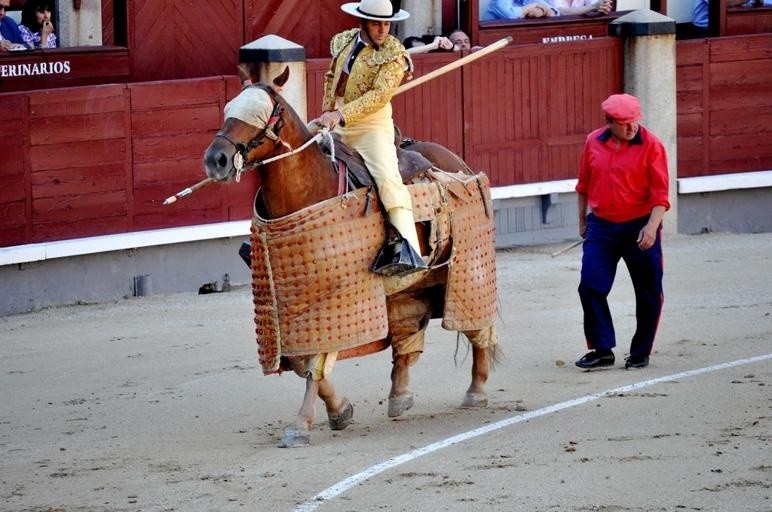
[602,92,644,125]
[340,0,413,24]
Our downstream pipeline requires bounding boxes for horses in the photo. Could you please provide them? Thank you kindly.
[201,65,503,448]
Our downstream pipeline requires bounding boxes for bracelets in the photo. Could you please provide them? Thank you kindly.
[449,44,455,52]
[534,6,545,15]
[39,43,48,48]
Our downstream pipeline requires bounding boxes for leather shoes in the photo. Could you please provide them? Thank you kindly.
[386,252,402,265]
[624,355,650,369]
[575,349,615,368]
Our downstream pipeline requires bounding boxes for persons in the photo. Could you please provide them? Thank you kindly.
[690,0,771,31]
[402,37,460,54]
[552,1,614,16]
[480,1,559,21]
[448,30,484,54]
[0,0,32,51]
[17,1,58,50]
[572,94,670,368]
[311,1,430,277]
